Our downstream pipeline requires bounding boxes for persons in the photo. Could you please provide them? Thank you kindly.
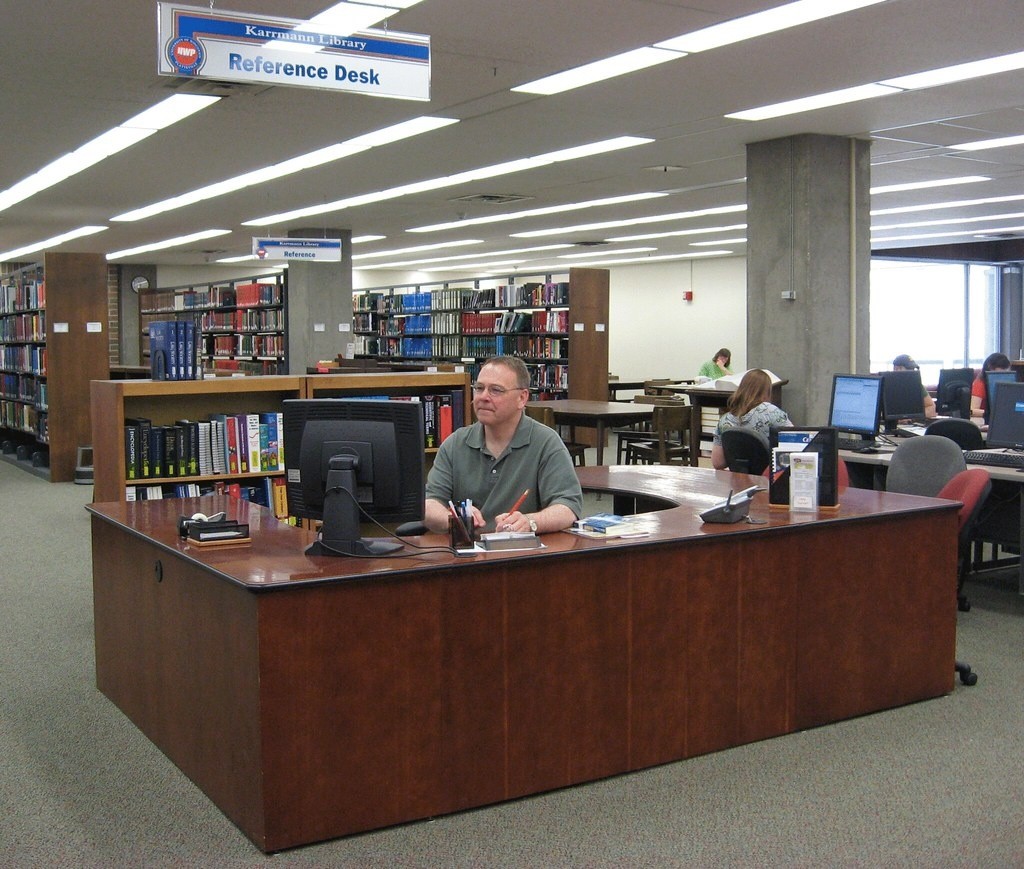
[699,348,734,380]
[970,352,1011,416]
[893,354,936,418]
[422,354,582,535]
[712,369,794,469]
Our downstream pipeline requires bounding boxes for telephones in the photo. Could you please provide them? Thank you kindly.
[700,484,764,523]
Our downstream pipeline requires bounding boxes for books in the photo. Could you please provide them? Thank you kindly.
[139,284,285,380]
[124,411,304,529]
[466,363,480,384]
[432,335,506,358]
[430,311,501,334]
[0,276,50,442]
[352,313,431,335]
[350,390,464,449]
[500,311,569,358]
[354,336,432,356]
[495,282,569,307]
[432,288,495,311]
[353,292,431,313]
[526,364,568,402]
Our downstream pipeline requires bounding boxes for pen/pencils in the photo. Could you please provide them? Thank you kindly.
[502,490,530,520]
[446,498,476,545]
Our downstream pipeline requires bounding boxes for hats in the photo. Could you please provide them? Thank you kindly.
[893,354,919,370]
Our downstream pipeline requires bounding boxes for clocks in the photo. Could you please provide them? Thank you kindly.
[131,276,147,295]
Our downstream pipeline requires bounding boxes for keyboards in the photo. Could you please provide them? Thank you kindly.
[963,452,1024,467]
[837,438,875,449]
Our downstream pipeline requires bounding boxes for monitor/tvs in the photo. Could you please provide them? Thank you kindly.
[984,370,1019,424]
[828,373,886,436]
[938,368,974,419]
[878,371,925,434]
[986,381,1024,450]
[282,399,426,558]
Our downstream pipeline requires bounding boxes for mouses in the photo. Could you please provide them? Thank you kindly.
[860,449,878,453]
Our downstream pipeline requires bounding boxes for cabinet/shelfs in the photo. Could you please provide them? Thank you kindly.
[0,250,111,486]
[350,263,614,448]
[139,275,288,378]
[87,372,468,529]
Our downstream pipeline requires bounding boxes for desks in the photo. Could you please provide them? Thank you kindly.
[526,396,674,501]
[836,402,1024,577]
[605,375,694,431]
[653,378,786,467]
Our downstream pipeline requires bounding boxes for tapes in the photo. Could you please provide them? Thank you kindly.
[191,509,225,524]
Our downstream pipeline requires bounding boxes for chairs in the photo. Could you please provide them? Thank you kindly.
[515,369,989,611]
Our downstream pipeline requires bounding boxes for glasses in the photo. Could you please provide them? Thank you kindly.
[472,383,524,397]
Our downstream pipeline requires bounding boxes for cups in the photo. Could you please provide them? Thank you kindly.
[448,514,474,550]
